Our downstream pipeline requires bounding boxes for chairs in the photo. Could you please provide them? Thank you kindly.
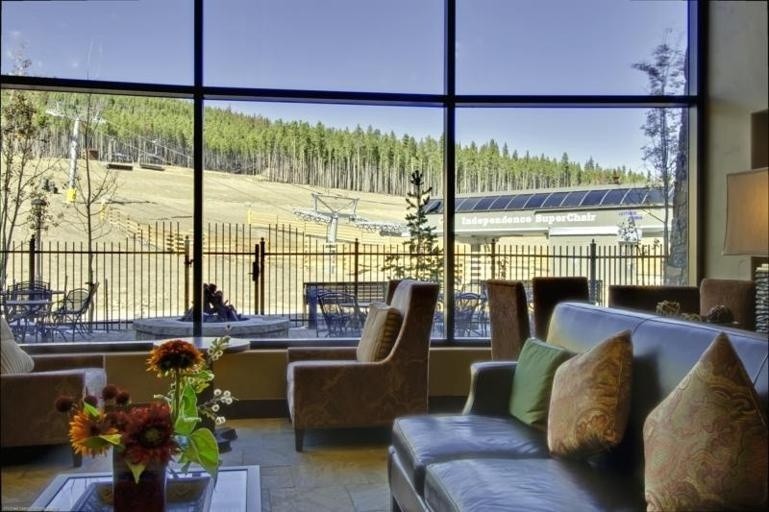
[0,296,112,476]
[283,275,440,457]
[476,271,755,367]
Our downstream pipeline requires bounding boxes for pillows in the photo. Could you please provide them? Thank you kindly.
[354,305,402,367]
[2,306,36,373]
[639,335,768,512]
[548,324,634,461]
[506,335,574,430]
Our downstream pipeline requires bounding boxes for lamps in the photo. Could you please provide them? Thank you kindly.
[722,163,769,334]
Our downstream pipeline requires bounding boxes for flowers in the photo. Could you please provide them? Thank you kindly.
[52,328,237,483]
[55,380,179,470]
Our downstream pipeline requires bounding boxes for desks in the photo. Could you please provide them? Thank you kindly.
[25,462,265,512]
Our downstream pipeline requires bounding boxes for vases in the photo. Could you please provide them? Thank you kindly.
[108,447,170,511]
[100,479,215,505]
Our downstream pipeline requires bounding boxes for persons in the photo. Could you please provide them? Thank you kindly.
[203,283,237,317]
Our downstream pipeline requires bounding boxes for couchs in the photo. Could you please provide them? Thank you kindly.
[383,299,769,512]
